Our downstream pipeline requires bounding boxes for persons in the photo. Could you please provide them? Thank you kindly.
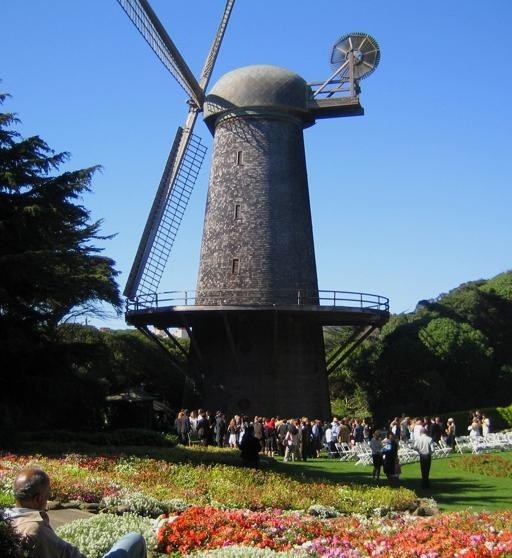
[467,409,491,438]
[0,467,147,558]
[157,407,456,488]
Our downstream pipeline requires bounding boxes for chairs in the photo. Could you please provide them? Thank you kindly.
[334,432,512,467]
